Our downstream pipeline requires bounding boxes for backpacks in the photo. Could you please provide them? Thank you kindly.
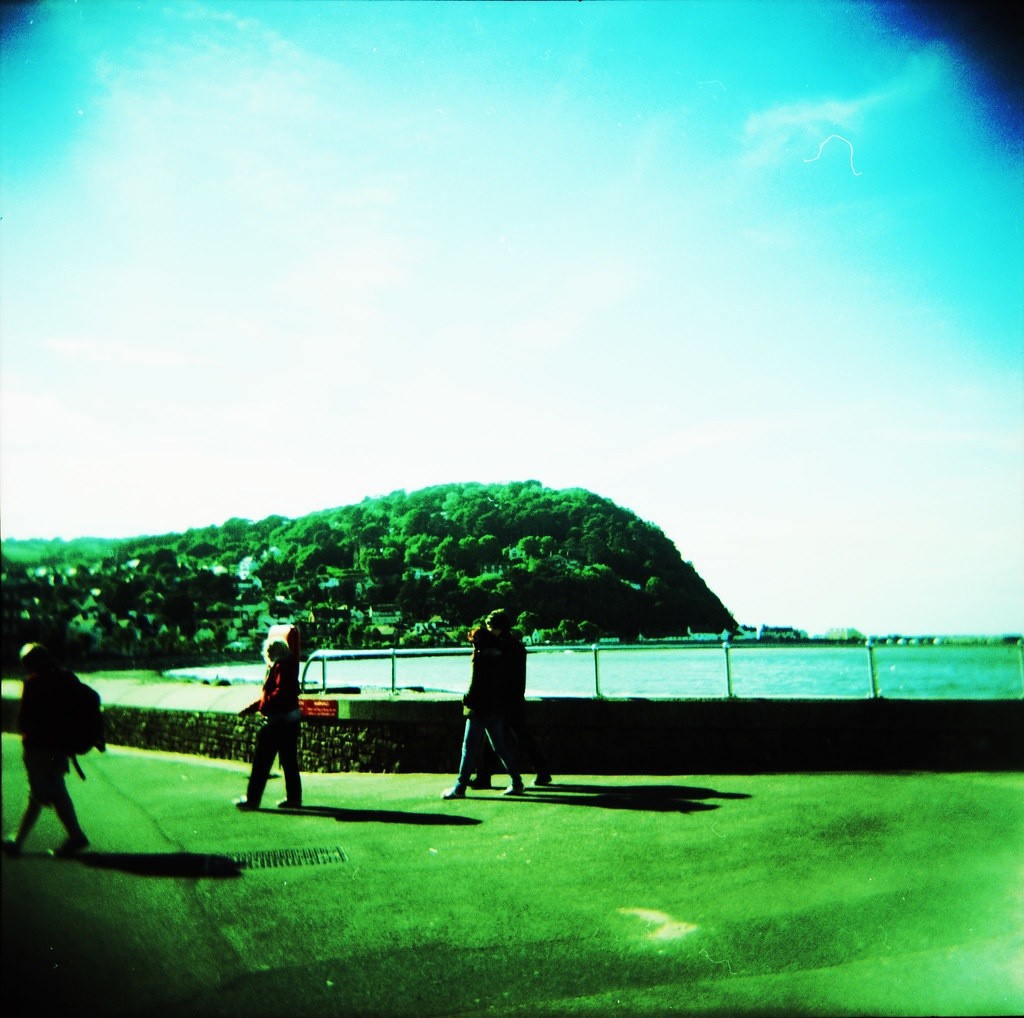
[35,668,106,780]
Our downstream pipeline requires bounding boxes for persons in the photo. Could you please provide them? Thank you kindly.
[1,642,90,856]
[468,608,551,789]
[233,637,304,809]
[442,624,523,800]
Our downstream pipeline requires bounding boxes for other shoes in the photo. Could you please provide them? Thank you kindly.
[443,792,466,799]
[534,772,552,786]
[471,778,492,790]
[53,833,90,859]
[236,802,258,812]
[278,801,303,809]
[504,784,523,795]
[2,839,21,861]
